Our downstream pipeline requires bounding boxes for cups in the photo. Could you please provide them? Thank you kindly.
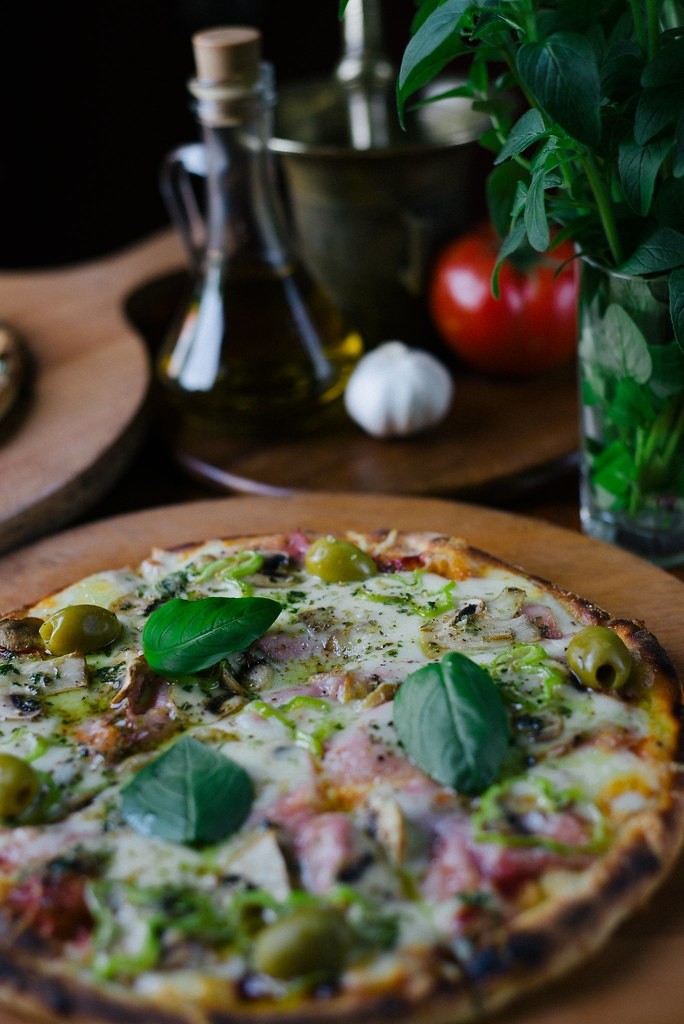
[235,75,505,321]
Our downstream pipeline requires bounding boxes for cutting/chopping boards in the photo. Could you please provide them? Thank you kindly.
[0,224,191,556]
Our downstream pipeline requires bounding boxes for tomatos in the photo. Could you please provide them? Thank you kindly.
[434,220,579,374]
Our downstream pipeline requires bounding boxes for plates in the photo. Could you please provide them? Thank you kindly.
[0,491,684,1024]
[142,379,577,498]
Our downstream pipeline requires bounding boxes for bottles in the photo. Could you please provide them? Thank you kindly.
[171,28,335,442]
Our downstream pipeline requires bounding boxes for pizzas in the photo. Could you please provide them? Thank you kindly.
[0,532,684,1023]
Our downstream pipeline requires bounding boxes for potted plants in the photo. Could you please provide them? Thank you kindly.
[392,0,684,572]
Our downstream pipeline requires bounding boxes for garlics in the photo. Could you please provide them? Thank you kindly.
[342,342,454,440]
